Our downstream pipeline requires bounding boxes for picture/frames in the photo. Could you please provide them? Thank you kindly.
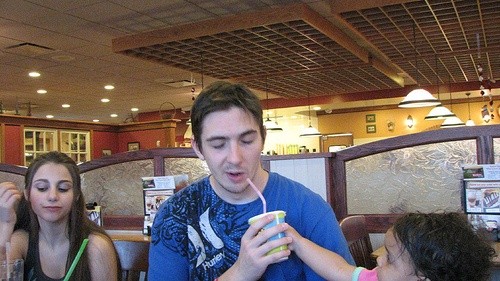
[127,142,140,152]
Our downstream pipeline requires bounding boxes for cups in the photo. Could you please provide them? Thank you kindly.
[248,211,288,264]
[0,259,24,281]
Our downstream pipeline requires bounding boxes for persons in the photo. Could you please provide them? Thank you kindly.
[283,208,497,280]
[146,79,357,281]
[0,151,123,280]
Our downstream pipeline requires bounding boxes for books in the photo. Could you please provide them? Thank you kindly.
[463,163,500,240]
[142,175,189,227]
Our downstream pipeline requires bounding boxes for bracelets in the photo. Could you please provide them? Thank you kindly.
[214,277,218,281]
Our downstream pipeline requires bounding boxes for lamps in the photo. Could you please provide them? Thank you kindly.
[183,81,195,138]
[299,126,322,138]
[396,25,477,129]
[260,117,283,131]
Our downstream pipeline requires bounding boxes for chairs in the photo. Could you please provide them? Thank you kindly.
[338,214,404,281]
[105,234,156,281]
